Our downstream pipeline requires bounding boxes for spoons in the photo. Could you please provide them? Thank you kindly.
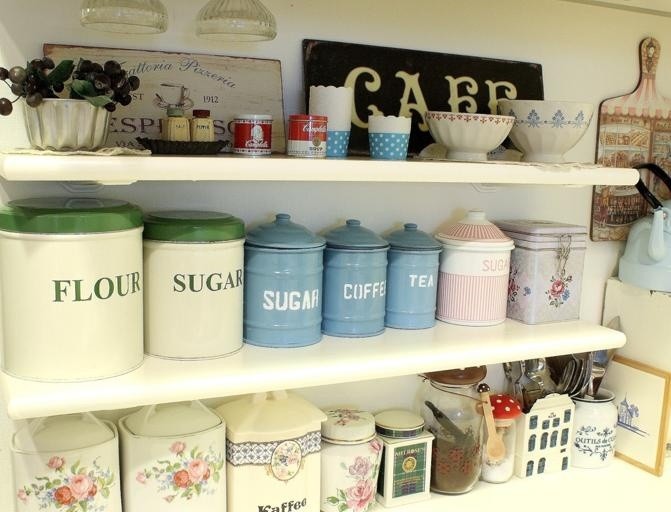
[503,353,593,416]
[482,392,505,460]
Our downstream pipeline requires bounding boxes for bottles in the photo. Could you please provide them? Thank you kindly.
[162,108,189,141]
[375,409,435,508]
[481,416,517,485]
[570,387,619,470]
[191,109,214,141]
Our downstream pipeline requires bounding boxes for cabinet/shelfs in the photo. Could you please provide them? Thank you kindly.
[0,142,642,511]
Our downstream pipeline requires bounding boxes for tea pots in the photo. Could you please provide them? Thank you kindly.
[616,164,671,293]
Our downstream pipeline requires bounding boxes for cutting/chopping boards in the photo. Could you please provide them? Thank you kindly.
[590,36,671,242]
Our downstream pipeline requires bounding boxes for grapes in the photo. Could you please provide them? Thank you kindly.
[1,56,63,115]
[71,60,139,112]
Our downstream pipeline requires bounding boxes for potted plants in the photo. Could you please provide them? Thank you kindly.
[1,55,142,152]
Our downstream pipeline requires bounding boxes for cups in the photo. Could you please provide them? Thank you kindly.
[367,113,412,160]
[307,83,353,158]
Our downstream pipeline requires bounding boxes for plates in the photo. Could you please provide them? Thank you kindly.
[135,136,229,157]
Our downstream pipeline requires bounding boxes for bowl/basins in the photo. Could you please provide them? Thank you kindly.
[497,99,595,165]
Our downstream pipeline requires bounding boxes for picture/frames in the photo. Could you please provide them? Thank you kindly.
[601,355,671,477]
